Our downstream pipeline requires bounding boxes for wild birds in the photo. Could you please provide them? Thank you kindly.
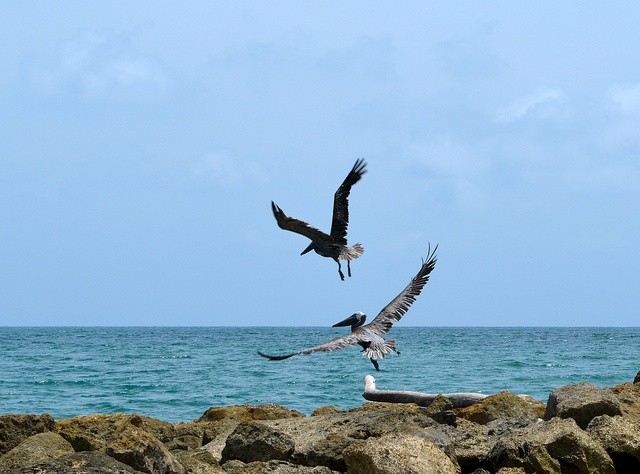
[256,239,439,372]
[270,156,367,281]
